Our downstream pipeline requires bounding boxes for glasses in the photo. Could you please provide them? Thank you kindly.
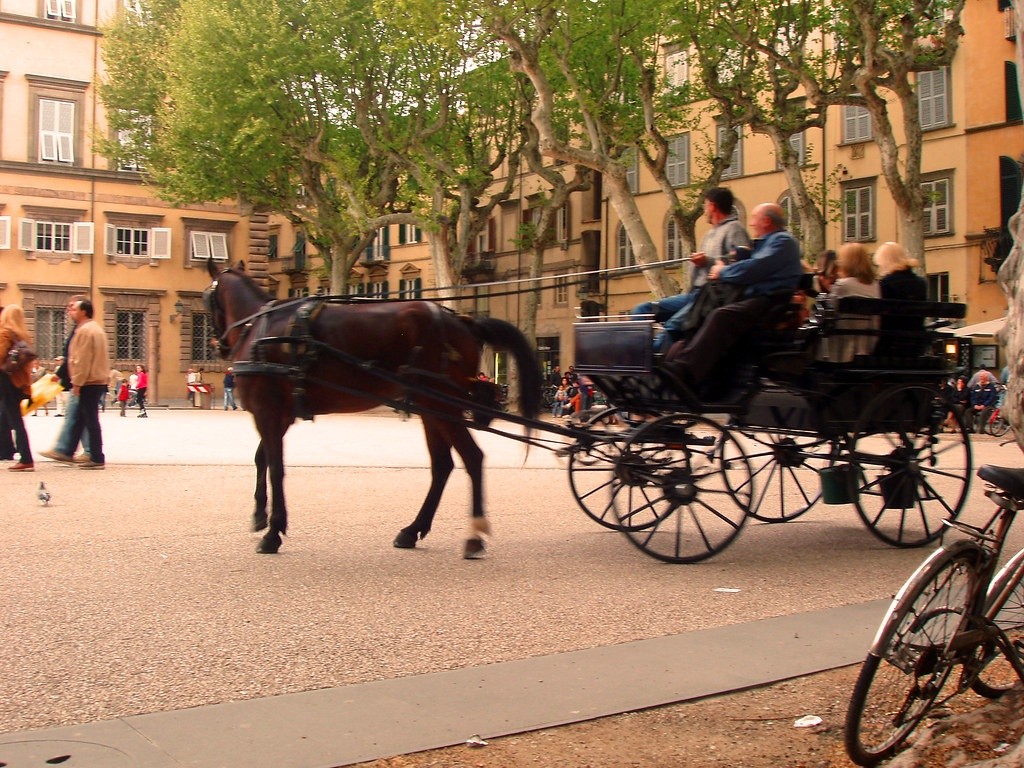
[701,202,714,210]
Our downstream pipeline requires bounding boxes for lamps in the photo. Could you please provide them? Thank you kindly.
[170,299,184,322]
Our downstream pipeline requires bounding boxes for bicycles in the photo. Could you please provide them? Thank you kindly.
[949,381,1014,436]
[838,464,1024,768]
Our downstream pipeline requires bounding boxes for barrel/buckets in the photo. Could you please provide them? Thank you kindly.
[818,446,863,506]
[876,459,919,510]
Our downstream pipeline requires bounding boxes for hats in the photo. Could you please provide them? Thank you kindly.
[54,355,65,361]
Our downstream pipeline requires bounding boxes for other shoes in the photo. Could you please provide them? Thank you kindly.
[557,414,562,418]
[55,414,64,417]
[966,429,975,434]
[137,409,148,418]
[552,415,555,418]
[233,406,237,410]
[951,430,956,433]
[942,423,948,427]
[8,462,35,471]
[74,455,92,463]
[979,429,984,434]
[40,450,73,462]
[78,459,106,469]
[562,405,569,410]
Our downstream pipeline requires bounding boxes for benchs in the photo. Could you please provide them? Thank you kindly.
[634,290,829,379]
[836,297,966,382]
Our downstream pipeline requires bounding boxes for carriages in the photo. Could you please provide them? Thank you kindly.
[201,255,975,565]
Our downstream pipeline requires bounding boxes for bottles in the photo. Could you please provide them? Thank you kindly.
[7,350,18,373]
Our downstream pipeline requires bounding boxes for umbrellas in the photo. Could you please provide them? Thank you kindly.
[953,316,1009,339]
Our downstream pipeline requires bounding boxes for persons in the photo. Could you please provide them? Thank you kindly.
[223,367,237,410]
[937,362,1009,433]
[186,368,204,400]
[550,365,655,425]
[475,369,508,403]
[623,184,927,387]
[31,295,109,470]
[108,365,147,418]
[0,303,34,471]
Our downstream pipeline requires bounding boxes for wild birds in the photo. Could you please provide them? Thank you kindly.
[38,481,50,505]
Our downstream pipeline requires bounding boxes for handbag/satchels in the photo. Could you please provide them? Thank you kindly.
[3,328,38,372]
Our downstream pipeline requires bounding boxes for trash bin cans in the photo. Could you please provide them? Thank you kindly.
[194,384,211,408]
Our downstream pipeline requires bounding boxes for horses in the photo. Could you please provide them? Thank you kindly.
[204,256,544,560]
